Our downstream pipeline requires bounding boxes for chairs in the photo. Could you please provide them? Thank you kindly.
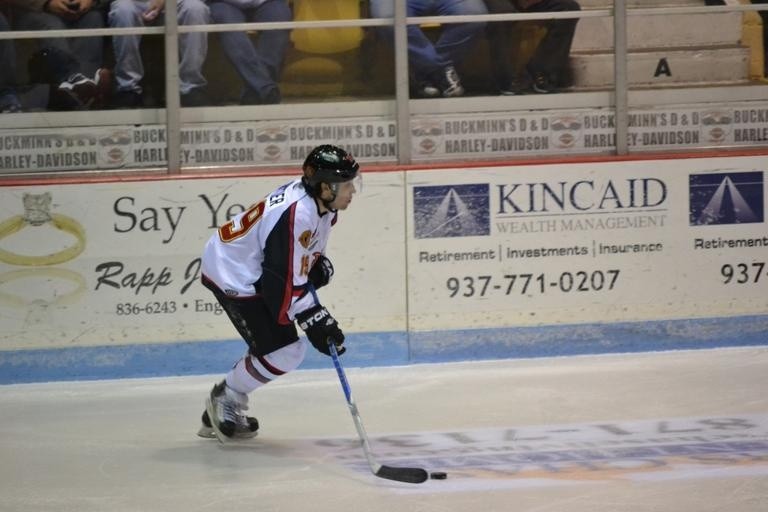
[279,1,396,103]
[424,23,550,90]
[205,31,258,100]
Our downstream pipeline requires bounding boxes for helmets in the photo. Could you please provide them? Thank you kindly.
[300,144,360,196]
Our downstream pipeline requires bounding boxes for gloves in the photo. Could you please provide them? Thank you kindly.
[296,305,346,356]
[308,256,335,290]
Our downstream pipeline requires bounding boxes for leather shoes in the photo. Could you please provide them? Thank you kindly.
[243,87,280,105]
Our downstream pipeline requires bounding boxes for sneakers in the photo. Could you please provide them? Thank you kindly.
[201,410,259,433]
[185,90,211,107]
[441,66,465,97]
[114,91,142,110]
[61,68,112,111]
[212,379,237,438]
[419,77,441,98]
[494,74,516,96]
[526,61,550,94]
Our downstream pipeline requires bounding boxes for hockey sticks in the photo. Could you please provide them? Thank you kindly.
[307,279,429,484]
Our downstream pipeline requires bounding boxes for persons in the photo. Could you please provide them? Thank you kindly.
[198,145,360,445]
